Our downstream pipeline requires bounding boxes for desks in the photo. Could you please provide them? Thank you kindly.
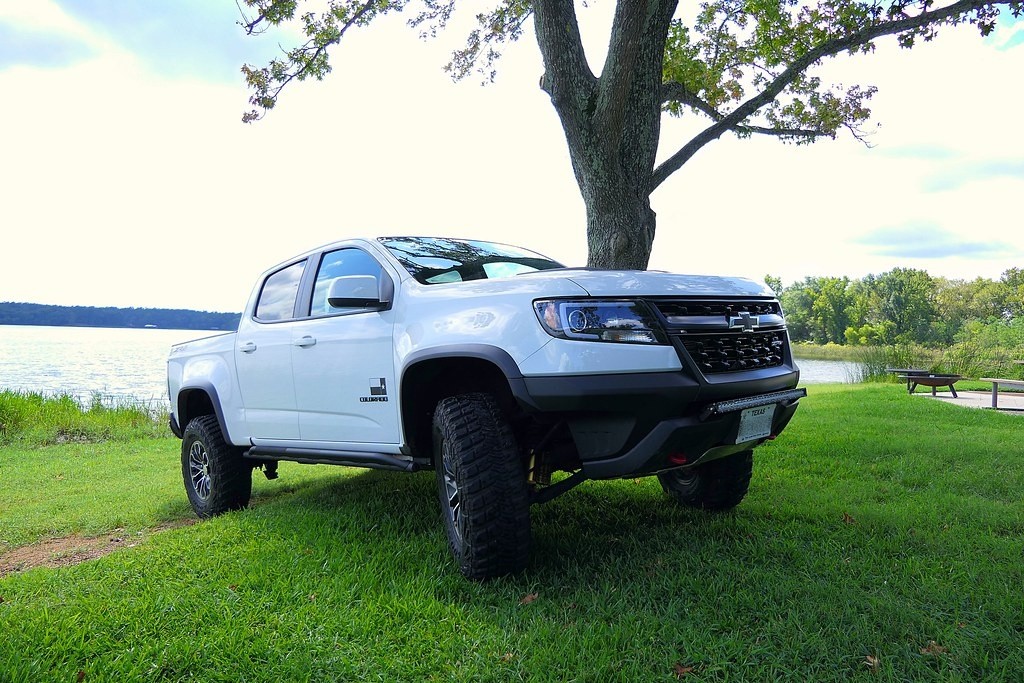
[884,369,929,390]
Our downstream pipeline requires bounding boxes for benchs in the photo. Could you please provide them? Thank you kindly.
[979,377,1024,410]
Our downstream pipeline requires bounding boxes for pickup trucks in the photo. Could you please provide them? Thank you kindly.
[165,234,808,581]
